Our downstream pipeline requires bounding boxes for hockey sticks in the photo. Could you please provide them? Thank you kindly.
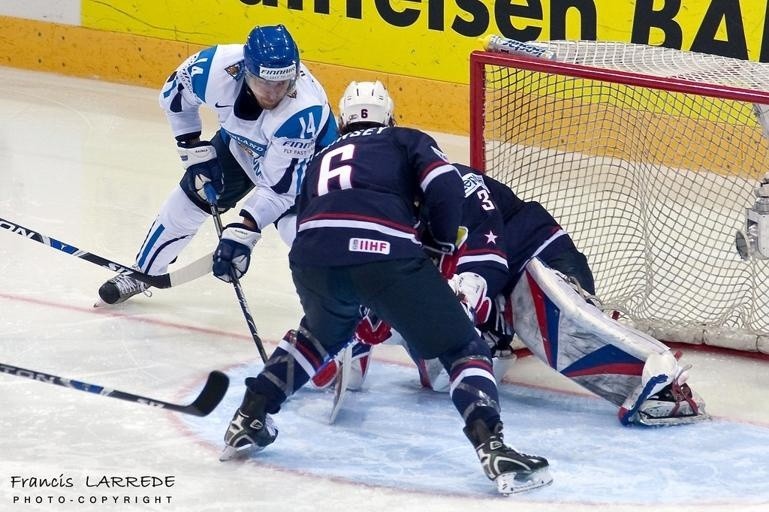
[0,362,229,417]
[0,218,216,289]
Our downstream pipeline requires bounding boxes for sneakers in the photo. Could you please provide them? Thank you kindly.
[98,263,153,304]
[462,418,549,481]
[642,385,706,418]
[223,388,279,448]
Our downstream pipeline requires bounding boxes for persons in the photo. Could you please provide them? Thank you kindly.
[221,78,549,480]
[96,24,339,306]
[302,160,706,425]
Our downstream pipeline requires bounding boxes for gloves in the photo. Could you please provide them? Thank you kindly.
[212,222,261,283]
[177,140,225,204]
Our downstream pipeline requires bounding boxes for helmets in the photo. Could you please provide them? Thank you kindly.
[242,24,299,99]
[337,80,394,125]
